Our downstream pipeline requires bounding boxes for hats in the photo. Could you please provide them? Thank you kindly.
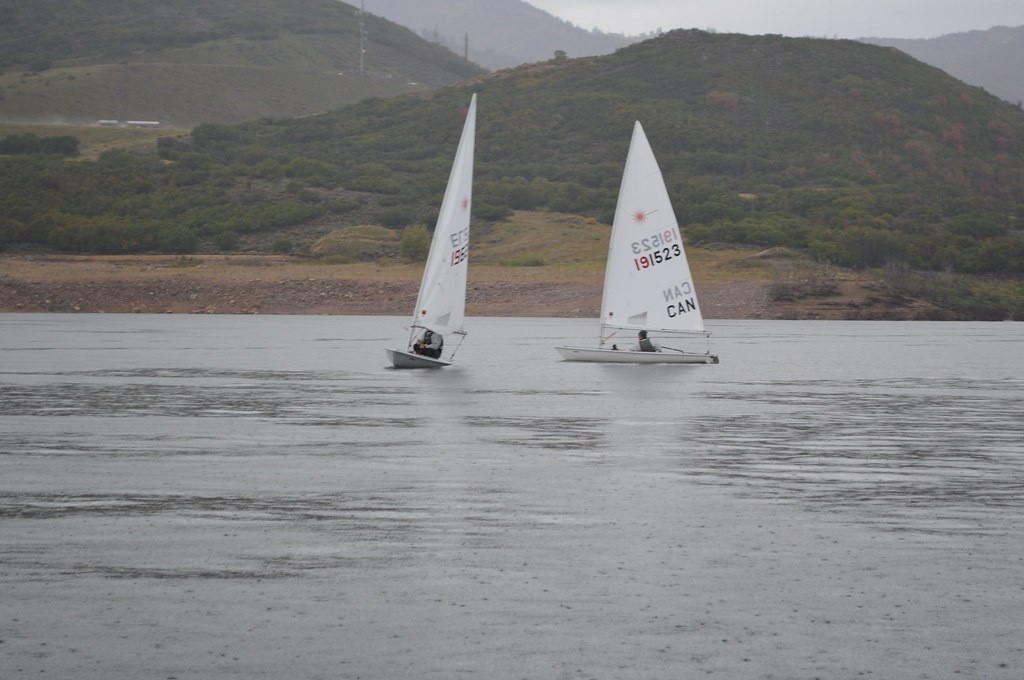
[639,330,647,338]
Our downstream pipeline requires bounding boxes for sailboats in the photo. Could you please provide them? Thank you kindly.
[554,121,721,365]
[383,91,478,368]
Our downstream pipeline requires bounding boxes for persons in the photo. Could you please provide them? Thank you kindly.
[414,331,443,359]
[626,331,661,352]
